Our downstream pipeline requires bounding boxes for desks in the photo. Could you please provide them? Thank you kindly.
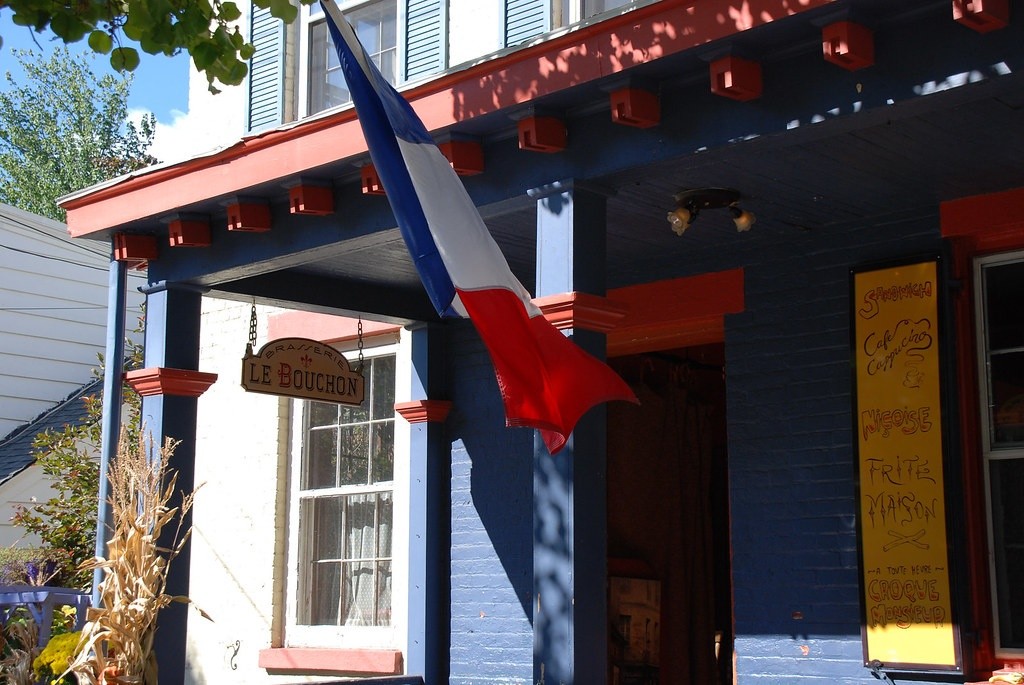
[0,585,90,647]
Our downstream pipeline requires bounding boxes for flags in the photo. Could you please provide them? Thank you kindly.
[320,0,643,453]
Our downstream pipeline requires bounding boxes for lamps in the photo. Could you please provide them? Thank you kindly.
[665,201,698,238]
[725,198,758,232]
